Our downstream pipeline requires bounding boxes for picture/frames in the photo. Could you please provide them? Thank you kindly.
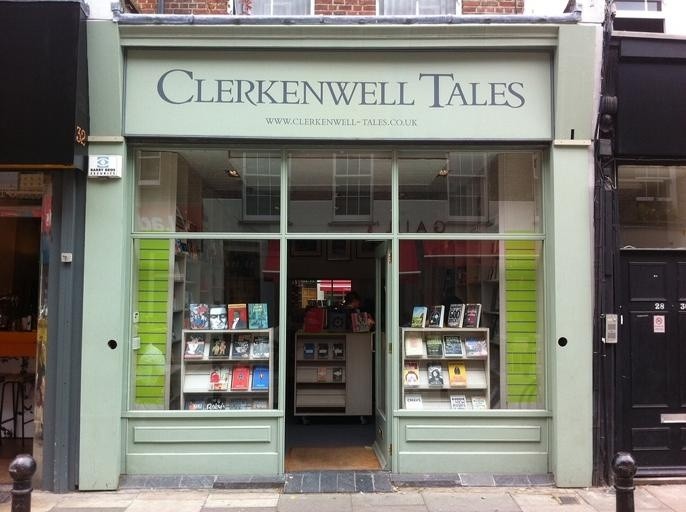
[288,239,376,262]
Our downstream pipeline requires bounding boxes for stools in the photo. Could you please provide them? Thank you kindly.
[1,373,36,449]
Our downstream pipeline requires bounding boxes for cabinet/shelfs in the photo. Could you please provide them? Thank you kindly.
[399,327,491,410]
[444,152,542,234]
[135,150,204,254]
[132,235,224,410]
[180,326,275,409]
[294,332,375,425]
[428,238,544,408]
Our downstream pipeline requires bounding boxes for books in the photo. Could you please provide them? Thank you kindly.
[182,299,271,410]
[404,301,489,412]
[300,301,372,383]
